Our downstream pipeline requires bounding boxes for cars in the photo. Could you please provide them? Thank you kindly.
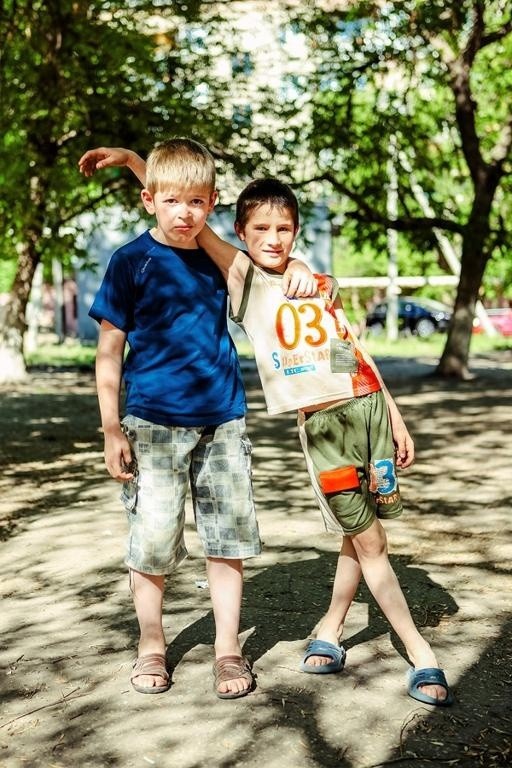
[368,299,512,340]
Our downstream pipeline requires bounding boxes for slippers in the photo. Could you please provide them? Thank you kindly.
[210,654,255,699]
[406,665,456,707]
[300,637,347,675]
[130,652,172,694]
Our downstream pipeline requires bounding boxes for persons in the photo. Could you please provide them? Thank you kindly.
[88,138,318,701]
[77,146,457,707]
